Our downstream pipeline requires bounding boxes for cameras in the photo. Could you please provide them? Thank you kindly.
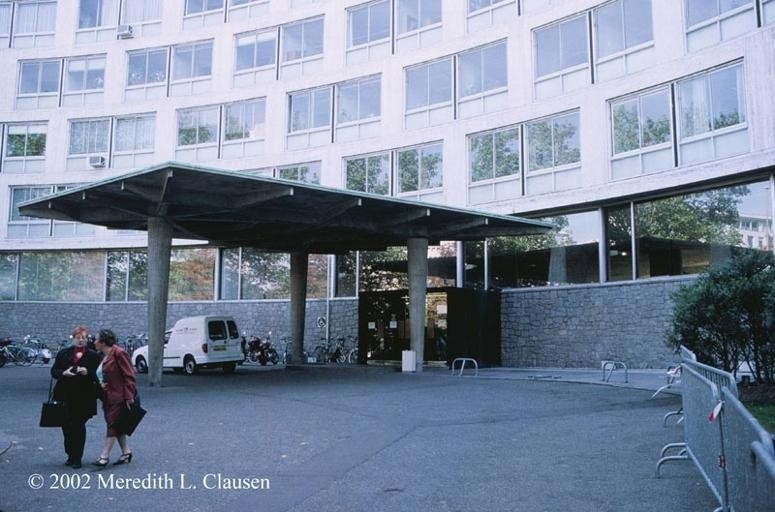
[70,366,81,375]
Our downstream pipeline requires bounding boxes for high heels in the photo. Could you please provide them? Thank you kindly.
[65,455,81,468]
[112,451,132,466]
[92,457,109,468]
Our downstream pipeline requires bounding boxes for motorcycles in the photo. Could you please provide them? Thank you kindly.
[238,331,279,366]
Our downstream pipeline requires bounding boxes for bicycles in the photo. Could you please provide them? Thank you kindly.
[0,333,148,367]
[280,335,362,364]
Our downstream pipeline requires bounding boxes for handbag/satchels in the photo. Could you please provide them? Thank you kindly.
[40,370,64,428]
[111,403,147,436]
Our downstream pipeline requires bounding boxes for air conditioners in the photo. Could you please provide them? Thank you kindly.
[118,24,135,39]
[91,156,107,168]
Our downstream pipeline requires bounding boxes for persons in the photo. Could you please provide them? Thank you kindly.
[92,329,136,466]
[51,327,101,468]
[0,333,170,363]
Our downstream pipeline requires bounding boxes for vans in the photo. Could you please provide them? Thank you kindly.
[131,314,245,374]
